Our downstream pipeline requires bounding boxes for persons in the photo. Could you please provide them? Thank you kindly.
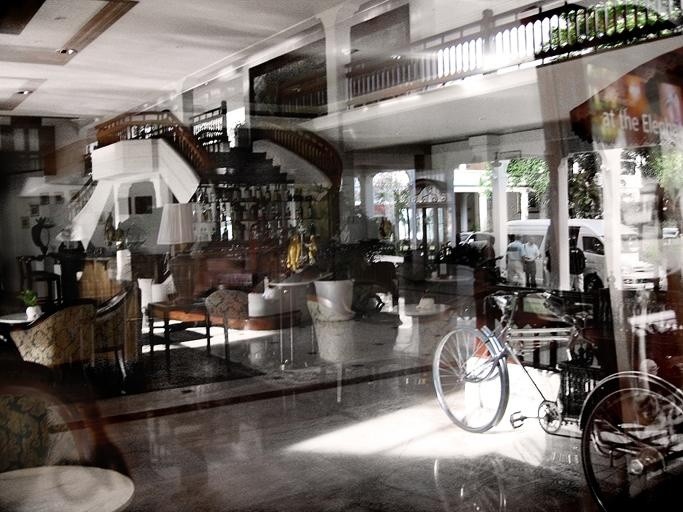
[520,237,539,287]
[480,236,496,260]
[504,234,526,287]
[568,236,586,292]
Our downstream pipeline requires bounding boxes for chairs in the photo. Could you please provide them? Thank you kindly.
[8,281,140,375]
[1,361,107,472]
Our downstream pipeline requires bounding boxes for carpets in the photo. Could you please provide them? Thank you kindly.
[52,330,266,401]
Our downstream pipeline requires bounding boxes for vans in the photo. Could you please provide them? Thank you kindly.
[460,220,661,290]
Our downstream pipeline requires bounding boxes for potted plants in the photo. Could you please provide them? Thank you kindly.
[306,233,390,317]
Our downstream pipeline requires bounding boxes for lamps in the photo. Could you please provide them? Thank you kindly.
[157,203,214,306]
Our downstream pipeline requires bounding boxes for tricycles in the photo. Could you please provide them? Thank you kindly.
[435,291,682,509]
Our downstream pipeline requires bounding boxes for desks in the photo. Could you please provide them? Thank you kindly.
[147,299,210,364]
[0,458,135,511]
[2,313,44,325]
